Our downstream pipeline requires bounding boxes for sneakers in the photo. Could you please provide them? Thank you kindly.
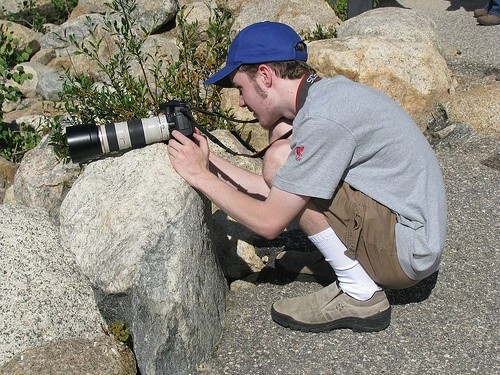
[277,249,342,283]
[271,281,391,334]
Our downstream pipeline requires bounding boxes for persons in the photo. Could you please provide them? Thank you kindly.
[473,0,500,25]
[167,21,447,333]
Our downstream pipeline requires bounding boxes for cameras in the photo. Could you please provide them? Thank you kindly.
[66,101,197,164]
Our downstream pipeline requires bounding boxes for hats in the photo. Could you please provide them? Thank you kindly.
[203,21,307,88]
